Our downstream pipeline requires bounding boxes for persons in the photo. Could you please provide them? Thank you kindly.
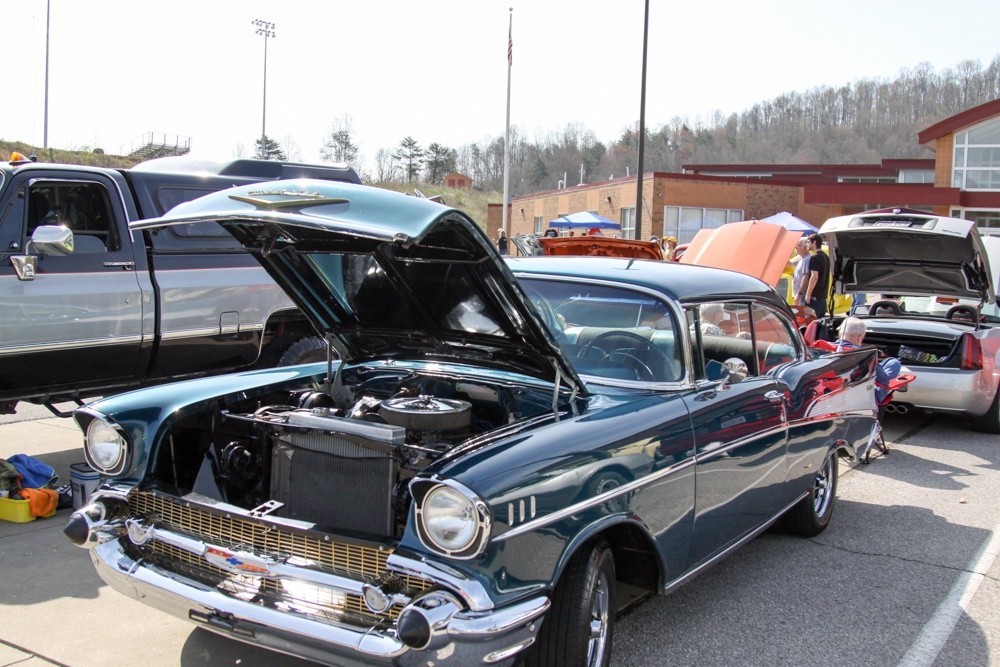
[496,228,508,256]
[544,227,586,237]
[688,303,726,337]
[786,233,901,403]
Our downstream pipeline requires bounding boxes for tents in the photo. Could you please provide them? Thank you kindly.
[548,211,621,239]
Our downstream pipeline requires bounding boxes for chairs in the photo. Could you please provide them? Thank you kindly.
[809,340,916,464]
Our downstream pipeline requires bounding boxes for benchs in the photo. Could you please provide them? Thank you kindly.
[565,326,796,383]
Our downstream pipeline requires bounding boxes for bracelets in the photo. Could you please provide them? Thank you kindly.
[797,291,803,295]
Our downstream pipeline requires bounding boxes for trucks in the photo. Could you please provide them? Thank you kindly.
[1,163,364,386]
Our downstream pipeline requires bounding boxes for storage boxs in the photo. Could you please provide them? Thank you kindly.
[0,498,36,524]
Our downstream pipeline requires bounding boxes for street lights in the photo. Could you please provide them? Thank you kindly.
[251,18,277,161]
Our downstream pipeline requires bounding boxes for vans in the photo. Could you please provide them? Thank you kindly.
[129,155,364,185]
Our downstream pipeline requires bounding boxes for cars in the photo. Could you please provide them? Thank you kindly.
[65,175,883,667]
[815,205,999,433]
